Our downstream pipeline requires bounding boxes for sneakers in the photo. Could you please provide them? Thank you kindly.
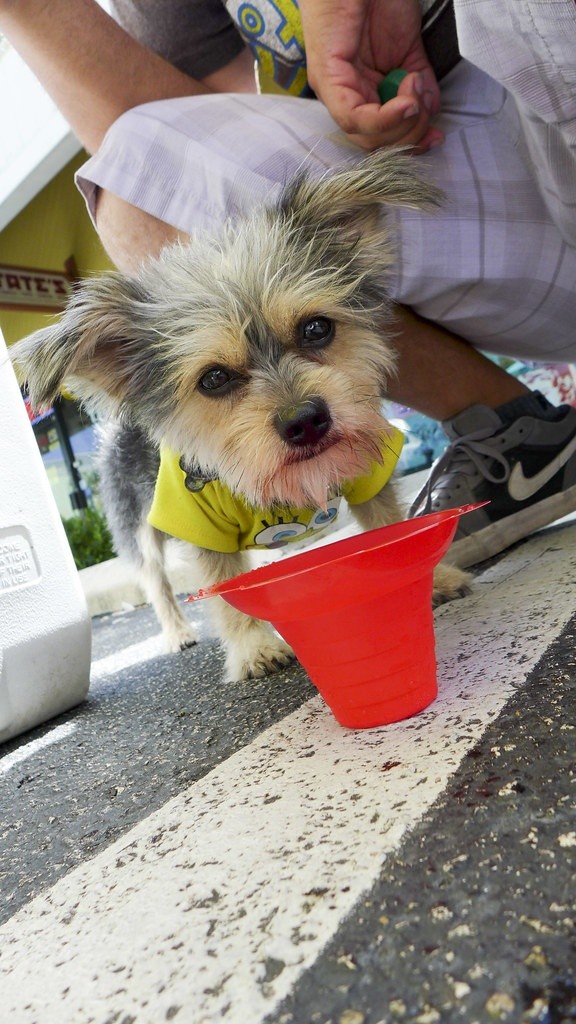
[406,402,576,570]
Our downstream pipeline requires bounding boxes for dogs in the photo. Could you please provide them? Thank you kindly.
[4,136,481,685]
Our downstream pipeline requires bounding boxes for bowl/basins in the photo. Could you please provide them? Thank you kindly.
[182,501,489,728]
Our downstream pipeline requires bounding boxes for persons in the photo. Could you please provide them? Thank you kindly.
[0,0,576,569]
